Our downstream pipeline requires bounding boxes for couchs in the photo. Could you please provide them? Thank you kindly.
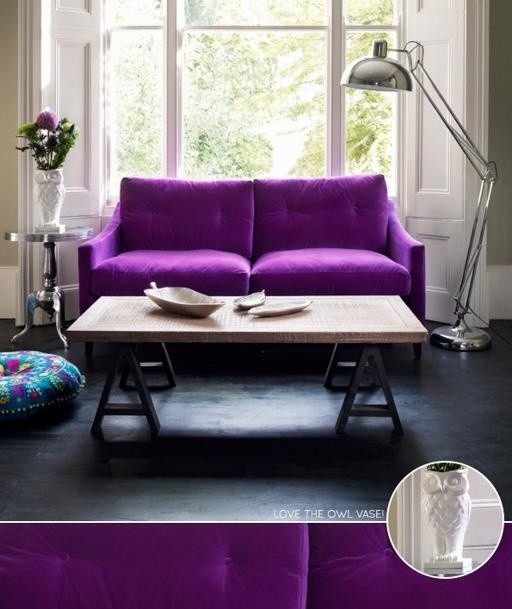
[77,171,427,361]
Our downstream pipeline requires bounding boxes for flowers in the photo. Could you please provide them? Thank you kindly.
[13,112,79,170]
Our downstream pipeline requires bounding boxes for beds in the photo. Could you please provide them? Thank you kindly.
[62,296,429,438]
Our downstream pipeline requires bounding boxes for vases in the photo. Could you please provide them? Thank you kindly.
[34,168,66,233]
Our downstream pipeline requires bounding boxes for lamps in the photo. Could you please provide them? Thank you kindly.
[337,38,497,352]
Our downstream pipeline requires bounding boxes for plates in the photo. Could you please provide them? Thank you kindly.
[144,282,312,317]
